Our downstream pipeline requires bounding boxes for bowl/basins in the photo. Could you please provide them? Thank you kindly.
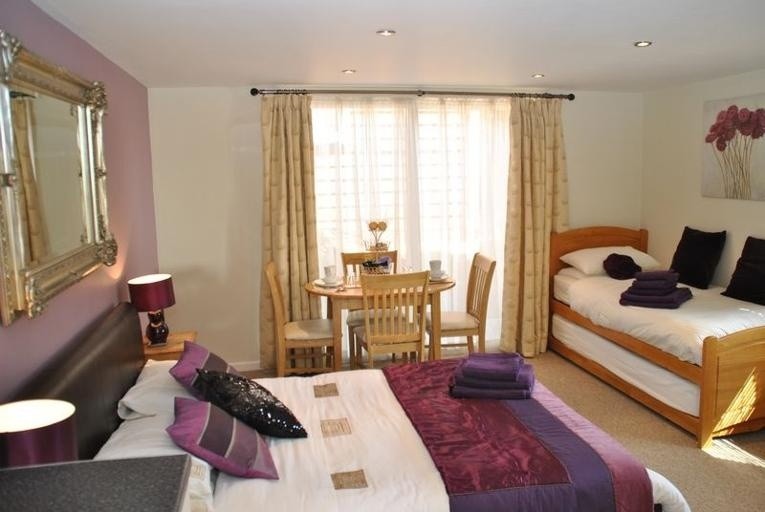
[430,270,447,279]
[322,277,340,284]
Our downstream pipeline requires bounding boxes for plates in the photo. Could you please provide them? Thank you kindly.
[428,273,452,282]
[315,279,344,287]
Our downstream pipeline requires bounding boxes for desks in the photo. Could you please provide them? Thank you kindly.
[1,453,192,512]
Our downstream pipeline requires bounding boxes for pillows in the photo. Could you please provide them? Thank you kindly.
[168,339,243,400]
[720,236,765,305]
[164,396,280,480]
[91,416,215,512]
[116,358,199,420]
[194,366,308,438]
[669,226,727,289]
[559,244,663,276]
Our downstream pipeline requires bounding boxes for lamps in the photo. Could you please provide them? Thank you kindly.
[126,274,177,344]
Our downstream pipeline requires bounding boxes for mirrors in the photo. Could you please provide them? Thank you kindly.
[0,28,119,327]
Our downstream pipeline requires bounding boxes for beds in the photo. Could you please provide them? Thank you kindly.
[547,226,765,448]
[14,299,694,512]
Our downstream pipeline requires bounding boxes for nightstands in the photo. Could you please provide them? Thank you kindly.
[141,329,199,359]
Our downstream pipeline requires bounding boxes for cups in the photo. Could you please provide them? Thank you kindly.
[430,260,441,273]
[324,266,336,278]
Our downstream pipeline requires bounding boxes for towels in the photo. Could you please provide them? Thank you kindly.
[603,253,642,280]
[454,355,536,390]
[626,276,678,295]
[462,351,522,381]
[634,268,675,281]
[449,376,537,399]
[620,288,692,309]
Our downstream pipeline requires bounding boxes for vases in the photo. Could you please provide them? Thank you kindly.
[360,255,392,274]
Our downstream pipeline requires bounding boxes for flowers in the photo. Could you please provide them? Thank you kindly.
[364,218,390,261]
[705,104,765,199]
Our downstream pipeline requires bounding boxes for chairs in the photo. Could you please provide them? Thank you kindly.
[419,252,497,360]
[354,270,431,368]
[341,250,409,371]
[265,261,344,378]
